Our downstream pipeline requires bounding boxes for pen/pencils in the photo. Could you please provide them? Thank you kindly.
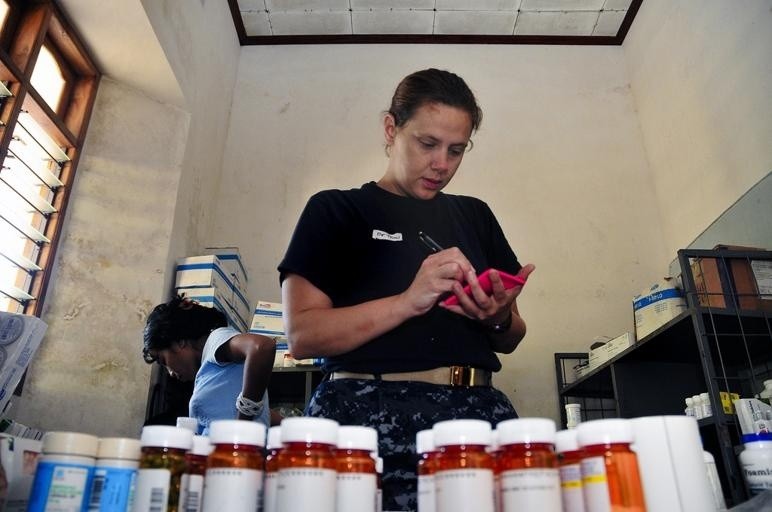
[417,231,444,253]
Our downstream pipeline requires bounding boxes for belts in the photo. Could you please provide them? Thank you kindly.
[328,362,494,389]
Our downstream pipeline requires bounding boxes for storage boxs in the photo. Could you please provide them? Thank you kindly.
[631,276,688,343]
[589,331,635,372]
[174,247,327,367]
[573,364,589,379]
[675,244,771,313]
[0,433,44,512]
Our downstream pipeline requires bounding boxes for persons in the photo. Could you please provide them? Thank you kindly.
[141,293,282,438]
[282,63,537,510]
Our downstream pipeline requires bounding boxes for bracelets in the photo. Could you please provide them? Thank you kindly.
[235,390,264,416]
[483,310,514,334]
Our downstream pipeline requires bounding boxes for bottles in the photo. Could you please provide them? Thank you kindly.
[26,415,386,511]
[684,393,710,419]
[739,433,771,492]
[565,403,583,428]
[415,415,647,512]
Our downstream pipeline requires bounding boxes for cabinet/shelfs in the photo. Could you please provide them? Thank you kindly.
[554,247,771,512]
